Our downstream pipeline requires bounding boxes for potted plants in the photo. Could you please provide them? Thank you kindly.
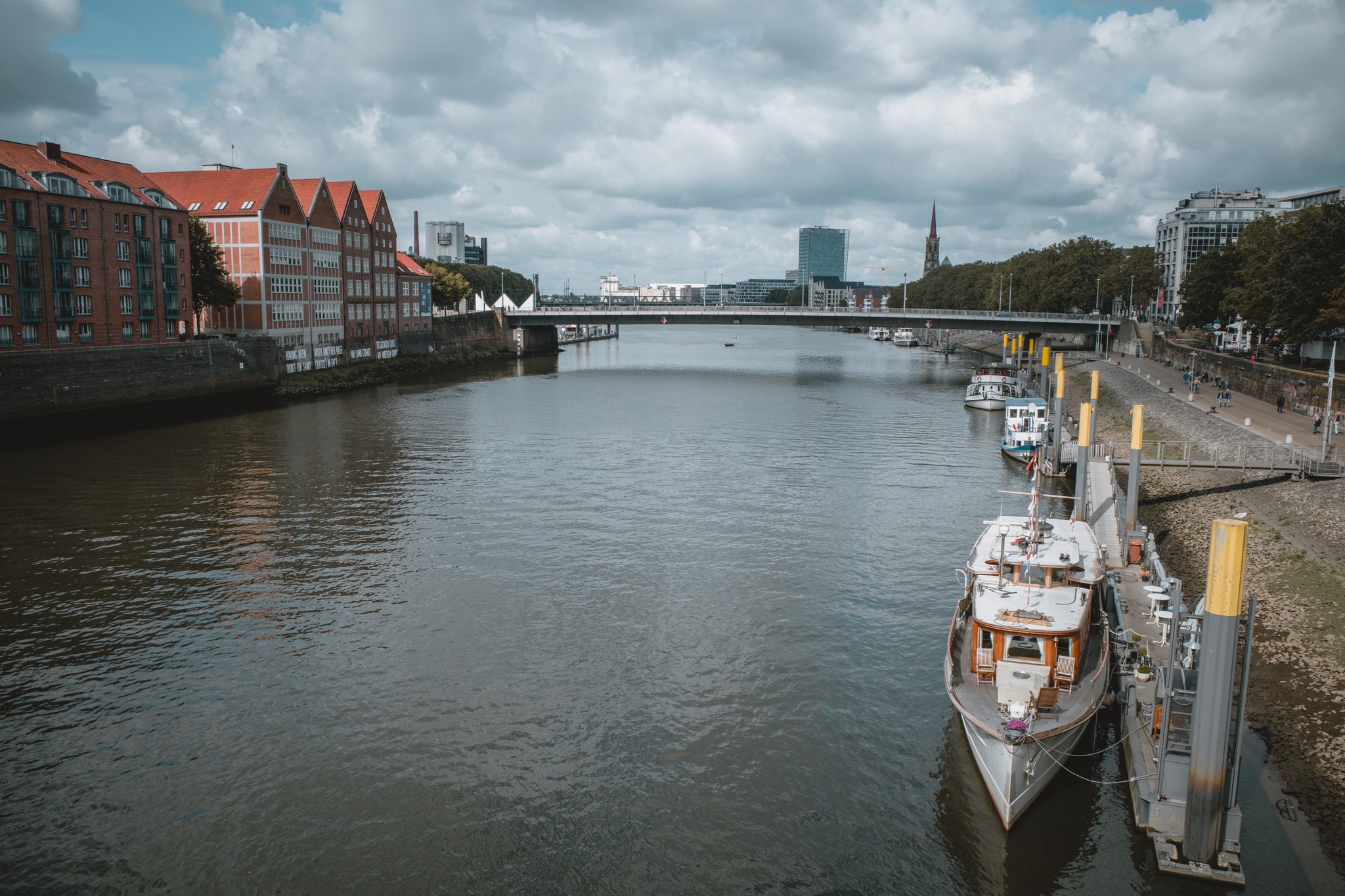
[1104,554,1110,572]
[1137,647,1151,682]
[1017,423,1022,432]
[1138,556,1149,579]
[1141,570,1148,582]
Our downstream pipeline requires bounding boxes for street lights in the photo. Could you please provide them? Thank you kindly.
[533,275,536,312]
[704,270,707,311]
[1096,279,1100,310]
[903,271,908,314]
[501,272,504,307]
[802,270,813,312]
[721,272,723,311]
[633,273,635,311]
[999,273,1012,318]
[1129,275,1135,319]
[609,271,611,311]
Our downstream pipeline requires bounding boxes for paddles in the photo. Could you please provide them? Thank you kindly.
[733,335,739,338]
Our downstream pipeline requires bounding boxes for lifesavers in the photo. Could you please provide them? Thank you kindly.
[978,376,982,382]
[1002,377,1006,383]
[939,343,943,346]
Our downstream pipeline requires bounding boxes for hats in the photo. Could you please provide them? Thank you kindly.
[1280,394,1283,396]
[1227,389,1229,391]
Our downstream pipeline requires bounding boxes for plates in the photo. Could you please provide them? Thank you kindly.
[1018,643,1033,648]
[1013,671,1030,679]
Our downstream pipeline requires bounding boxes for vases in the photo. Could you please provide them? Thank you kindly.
[1006,728,1025,736]
[1133,642,1140,649]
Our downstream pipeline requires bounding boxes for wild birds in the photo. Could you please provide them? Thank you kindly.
[1232,512,1249,521]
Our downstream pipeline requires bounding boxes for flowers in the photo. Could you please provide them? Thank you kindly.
[1007,719,1026,731]
[1132,635,1142,645]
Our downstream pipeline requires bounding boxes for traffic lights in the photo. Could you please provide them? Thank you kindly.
[1138,306,1141,310]
[1142,306,1143,310]
[1124,306,1126,310]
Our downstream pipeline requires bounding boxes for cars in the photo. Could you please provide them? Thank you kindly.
[553,301,765,303]
[772,302,776,304]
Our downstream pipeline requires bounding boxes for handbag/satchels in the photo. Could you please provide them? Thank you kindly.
[1183,376,1184,378]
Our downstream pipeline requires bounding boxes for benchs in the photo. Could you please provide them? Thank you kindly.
[1153,356,1229,390]
[1190,343,1264,362]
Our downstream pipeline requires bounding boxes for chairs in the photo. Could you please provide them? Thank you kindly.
[1280,346,1284,353]
[1028,655,1076,721]
[1037,424,1045,432]
[975,648,997,686]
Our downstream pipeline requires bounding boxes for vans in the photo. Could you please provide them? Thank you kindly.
[996,310,1013,317]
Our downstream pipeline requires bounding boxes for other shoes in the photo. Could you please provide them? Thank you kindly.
[1333,432,1335,433]
[1313,432,1316,434]
[1336,434,1338,435]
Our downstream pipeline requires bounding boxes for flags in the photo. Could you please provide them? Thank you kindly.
[1108,320,1112,335]
[1328,345,1335,384]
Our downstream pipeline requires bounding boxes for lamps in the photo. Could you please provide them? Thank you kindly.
[1238,367,1244,370]
[1155,344,1222,364]
[1297,381,1306,384]
[1018,539,1029,556]
[1052,635,1060,647]
[1264,373,1271,376]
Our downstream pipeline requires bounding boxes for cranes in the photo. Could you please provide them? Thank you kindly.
[847,265,894,286]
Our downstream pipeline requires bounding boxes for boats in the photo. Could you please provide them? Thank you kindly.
[942,444,1111,834]
[840,326,917,347]
[558,325,603,340]
[964,362,1022,411]
[1001,399,1049,465]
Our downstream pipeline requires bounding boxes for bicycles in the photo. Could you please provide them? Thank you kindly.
[857,308,864,313]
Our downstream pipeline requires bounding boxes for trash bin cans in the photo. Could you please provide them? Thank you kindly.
[1128,539,1143,565]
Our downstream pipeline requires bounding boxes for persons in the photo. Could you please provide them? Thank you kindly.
[1183,365,1196,387]
[493,305,515,312]
[1061,335,1063,343]
[1213,345,1256,361]
[1223,389,1232,408]
[1020,309,1084,319]
[1194,377,1201,392]
[1088,312,1093,320]
[1159,315,1173,326]
[1276,394,1285,414]
[1332,411,1343,435]
[1217,379,1225,390]
[1137,313,1147,323]
[716,303,718,310]
[1163,359,1171,367]
[720,304,724,311]
[1211,376,1221,387]
[819,303,834,313]
[859,304,862,310]
[1121,352,1125,358]
[1176,307,1181,319]
[1217,390,1224,408]
[1106,313,1110,320]
[1200,371,1208,383]
[613,304,619,310]
[1313,411,1320,434]
[536,304,605,311]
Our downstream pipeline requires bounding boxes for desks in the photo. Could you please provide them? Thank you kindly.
[1307,368,1329,375]
[1206,347,1212,351]
[1142,586,1173,647]
[1272,348,1281,361]
